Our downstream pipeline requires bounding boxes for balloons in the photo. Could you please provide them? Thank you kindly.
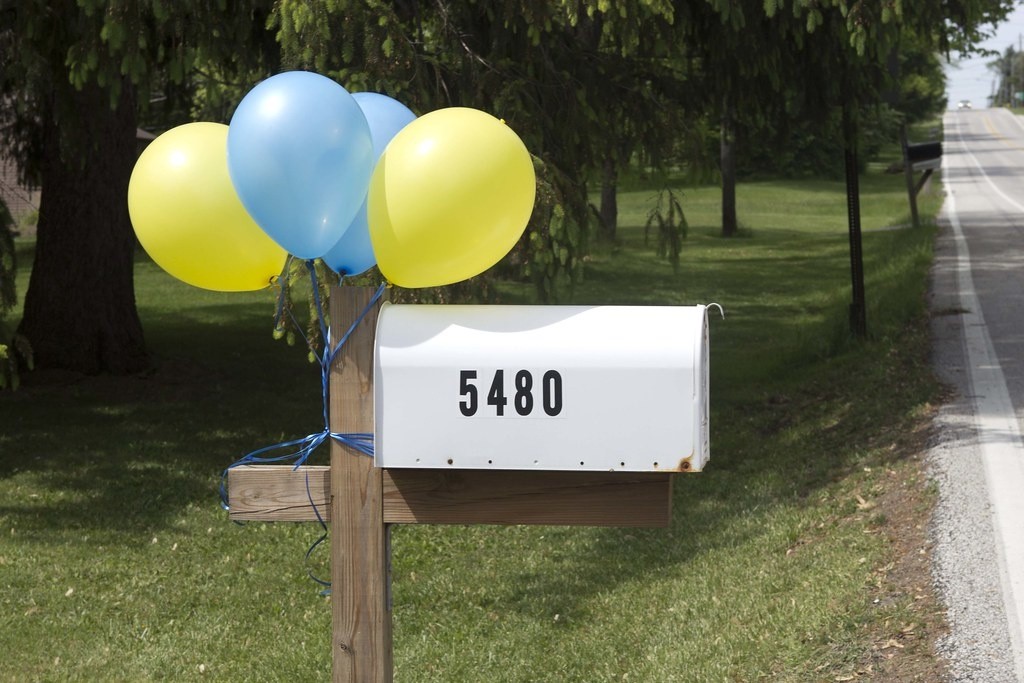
[227,71,374,261]
[367,107,536,290]
[323,91,417,277]
[127,122,291,292]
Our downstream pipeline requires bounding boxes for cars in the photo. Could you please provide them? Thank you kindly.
[958,99,972,111]
